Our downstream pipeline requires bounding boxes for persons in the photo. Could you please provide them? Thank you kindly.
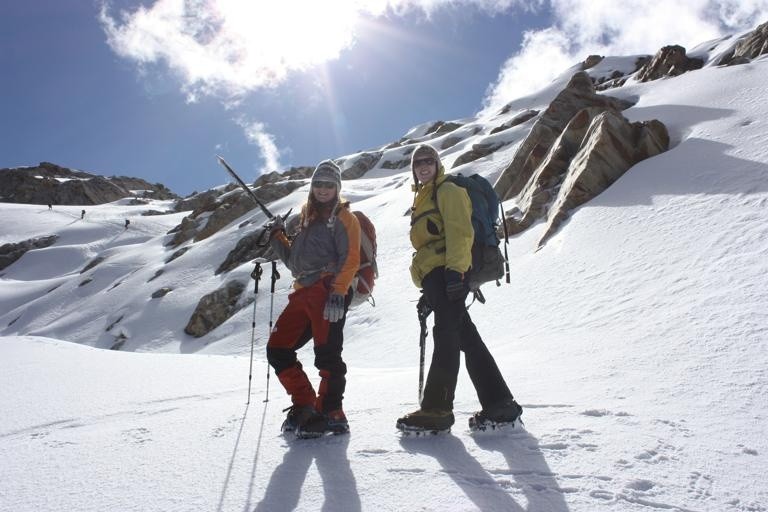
[266,160,361,439]
[397,145,523,435]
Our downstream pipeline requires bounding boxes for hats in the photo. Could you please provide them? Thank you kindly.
[412,145,440,183]
[309,159,341,199]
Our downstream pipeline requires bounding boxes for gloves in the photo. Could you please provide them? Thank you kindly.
[323,294,345,323]
[263,214,286,230]
[444,270,464,301]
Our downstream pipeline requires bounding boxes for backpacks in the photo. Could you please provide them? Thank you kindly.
[431,173,510,307]
[328,202,379,307]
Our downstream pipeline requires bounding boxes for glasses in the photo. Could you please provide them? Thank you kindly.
[414,158,435,165]
[313,181,334,188]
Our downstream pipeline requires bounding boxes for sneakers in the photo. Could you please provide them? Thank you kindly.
[283,405,349,438]
[397,401,522,430]
